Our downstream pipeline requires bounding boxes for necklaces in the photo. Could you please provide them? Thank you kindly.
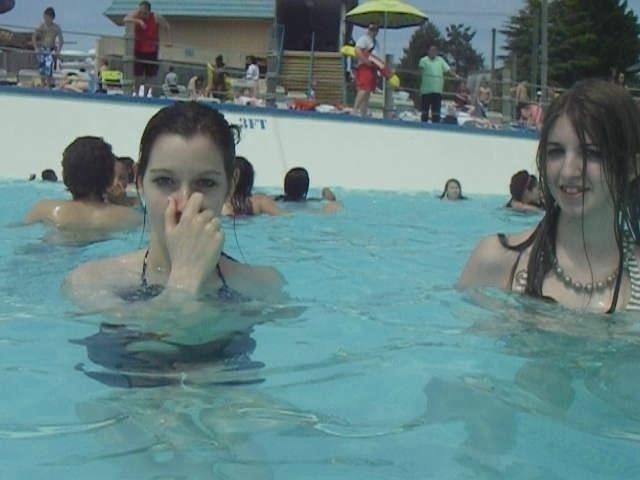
[546,229,640,311]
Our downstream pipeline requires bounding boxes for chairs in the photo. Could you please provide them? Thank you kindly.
[41,57,519,137]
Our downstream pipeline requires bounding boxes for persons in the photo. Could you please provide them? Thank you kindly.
[353,22,381,118]
[223,156,342,218]
[613,72,628,89]
[418,45,460,122]
[454,78,640,316]
[439,178,467,200]
[63,97,283,324]
[507,170,544,214]
[457,75,543,130]
[20,137,143,246]
[32,1,260,100]
[274,166,333,202]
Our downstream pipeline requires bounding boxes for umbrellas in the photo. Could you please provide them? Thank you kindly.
[344,0,427,108]
